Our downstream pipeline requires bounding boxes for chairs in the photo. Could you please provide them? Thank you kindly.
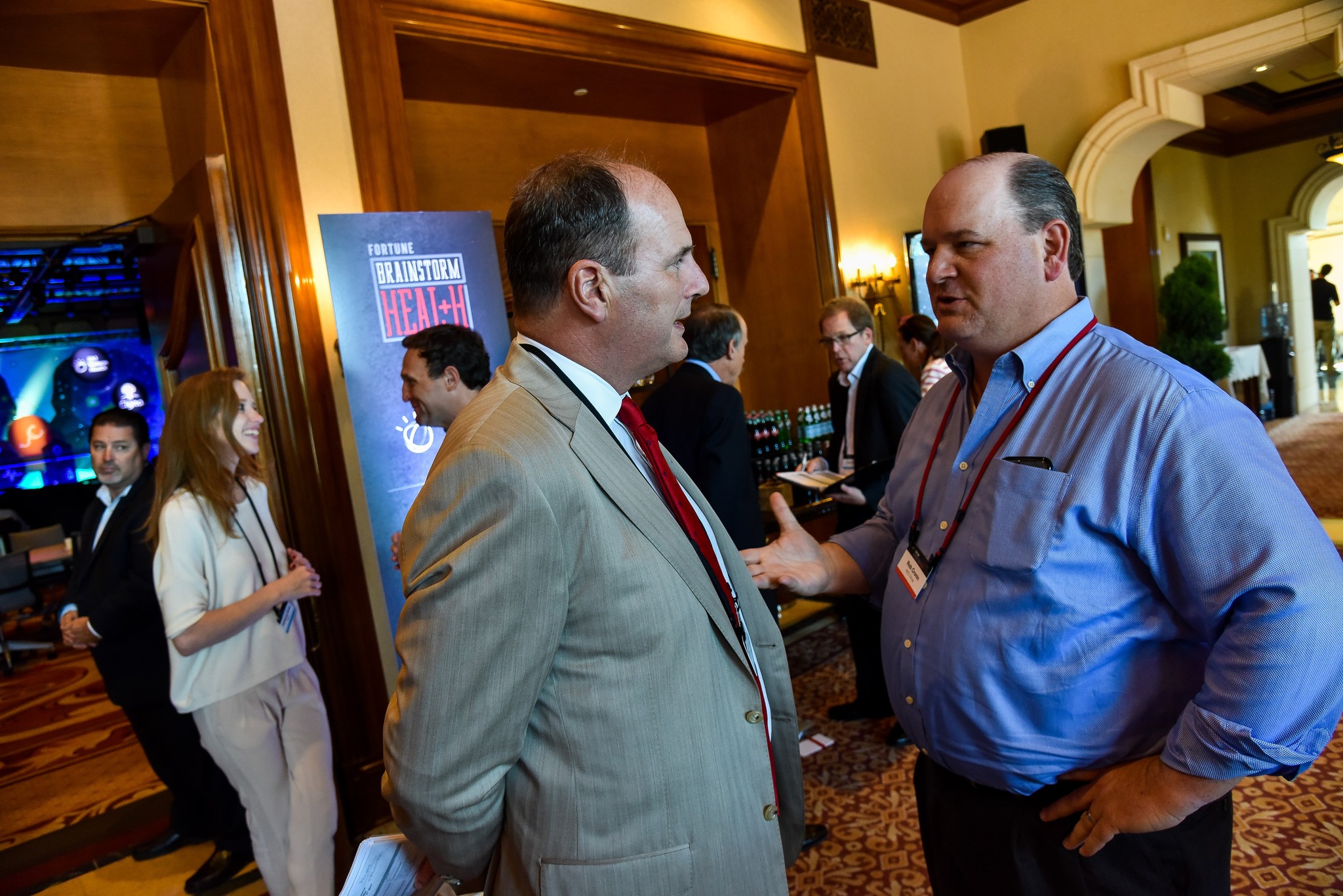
[0,555,55,674]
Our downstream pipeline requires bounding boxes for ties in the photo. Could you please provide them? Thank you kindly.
[617,395,780,817]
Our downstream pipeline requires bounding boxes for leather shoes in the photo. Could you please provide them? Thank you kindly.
[828,695,896,720]
[798,823,830,851]
[133,826,190,860]
[886,722,915,747]
[185,844,256,892]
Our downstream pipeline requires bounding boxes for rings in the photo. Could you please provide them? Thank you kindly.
[1086,809,1097,825]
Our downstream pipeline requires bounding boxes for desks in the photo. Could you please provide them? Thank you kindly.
[1215,345,1271,413]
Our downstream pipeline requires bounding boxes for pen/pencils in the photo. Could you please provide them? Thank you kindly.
[801,452,808,471]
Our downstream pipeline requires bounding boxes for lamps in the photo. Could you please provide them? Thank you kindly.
[838,246,902,306]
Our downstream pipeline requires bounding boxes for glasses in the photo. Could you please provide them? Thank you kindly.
[817,330,860,349]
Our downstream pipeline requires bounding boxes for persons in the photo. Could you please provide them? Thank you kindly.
[138,367,337,896]
[1310,264,1340,377]
[53,410,254,896]
[380,155,805,896]
[640,303,830,850]
[391,323,493,571]
[738,153,1343,896]
[796,295,952,748]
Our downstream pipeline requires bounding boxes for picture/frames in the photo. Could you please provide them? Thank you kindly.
[1180,232,1228,319]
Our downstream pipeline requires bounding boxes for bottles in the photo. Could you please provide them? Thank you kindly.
[742,401,833,527]
[1261,302,1289,338]
